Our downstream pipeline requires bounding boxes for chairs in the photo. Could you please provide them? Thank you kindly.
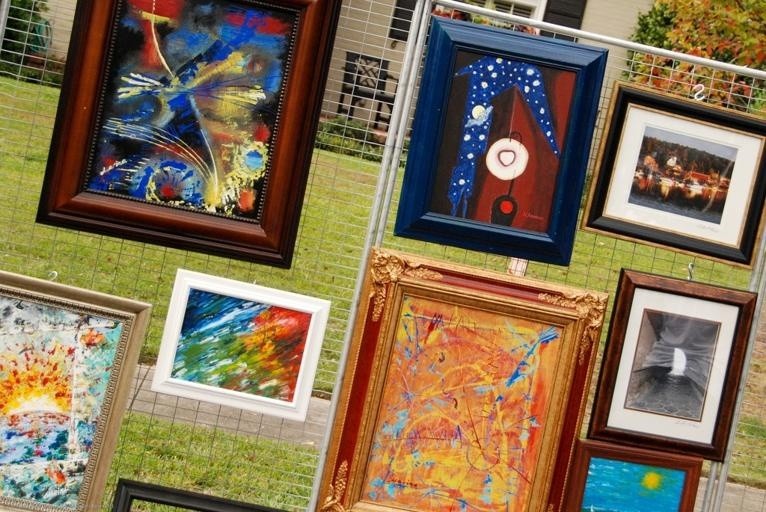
[335,50,399,131]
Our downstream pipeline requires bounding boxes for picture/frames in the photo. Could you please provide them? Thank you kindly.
[0,268,152,512]
[562,438,704,512]
[588,268,757,462]
[111,478,287,512]
[392,11,609,267]
[315,247,608,512]
[33,0,343,270]
[580,77,766,270]
[151,268,331,423]
